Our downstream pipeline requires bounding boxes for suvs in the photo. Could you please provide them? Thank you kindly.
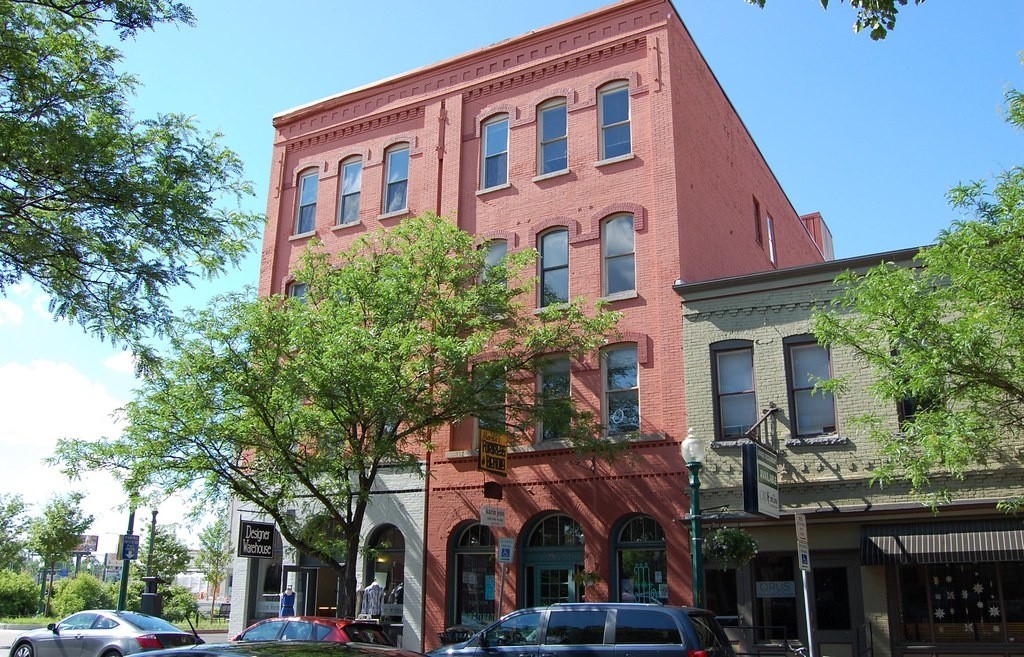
[422,601,735,657]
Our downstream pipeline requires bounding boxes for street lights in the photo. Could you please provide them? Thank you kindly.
[146,498,160,575]
[680,426,707,608]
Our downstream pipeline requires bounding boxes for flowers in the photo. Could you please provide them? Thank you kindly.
[702,526,759,572]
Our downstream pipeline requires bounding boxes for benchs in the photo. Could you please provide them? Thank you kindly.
[214,604,231,624]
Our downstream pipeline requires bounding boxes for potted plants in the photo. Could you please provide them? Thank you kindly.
[575,571,601,586]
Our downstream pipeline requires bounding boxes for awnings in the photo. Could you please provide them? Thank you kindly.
[860,517,1024,566]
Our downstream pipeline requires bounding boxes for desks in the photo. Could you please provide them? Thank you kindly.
[446,625,513,645]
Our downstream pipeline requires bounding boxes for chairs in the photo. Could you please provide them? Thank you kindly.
[102,621,109,627]
[285,624,296,639]
[437,631,469,644]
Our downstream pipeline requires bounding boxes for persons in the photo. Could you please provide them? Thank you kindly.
[388,582,403,605]
[280,585,297,617]
[361,582,386,621]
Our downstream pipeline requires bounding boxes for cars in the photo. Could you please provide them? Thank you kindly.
[6,609,207,657]
[117,640,432,657]
[228,615,397,648]
